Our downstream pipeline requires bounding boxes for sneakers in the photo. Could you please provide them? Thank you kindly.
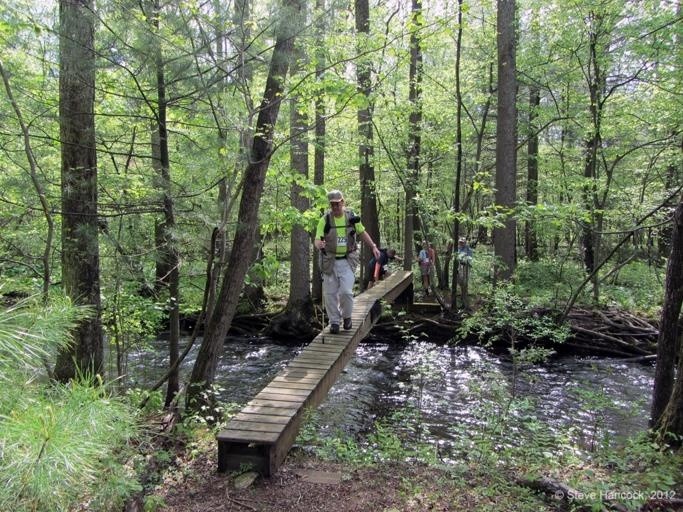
[342,316,353,330]
[329,323,340,335]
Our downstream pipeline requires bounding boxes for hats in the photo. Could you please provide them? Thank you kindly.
[326,190,344,204]
[458,236,466,243]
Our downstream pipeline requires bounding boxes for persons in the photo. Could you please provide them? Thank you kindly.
[357,247,405,291]
[312,189,380,334]
[417,240,435,296]
[455,237,473,300]
